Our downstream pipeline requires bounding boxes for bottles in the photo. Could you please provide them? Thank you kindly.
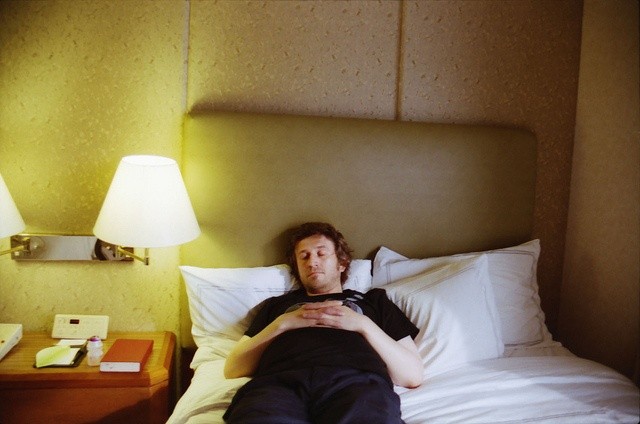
[86,337,103,366]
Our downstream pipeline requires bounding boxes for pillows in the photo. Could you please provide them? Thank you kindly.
[376,253,504,393]
[372,239,562,349]
[179,259,372,369]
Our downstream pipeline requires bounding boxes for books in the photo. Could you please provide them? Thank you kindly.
[99,339,154,373]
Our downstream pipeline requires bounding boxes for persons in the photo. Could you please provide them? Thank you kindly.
[223,222,424,424]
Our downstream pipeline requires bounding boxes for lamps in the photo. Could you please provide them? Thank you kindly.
[93,155,202,267]
[0,172,30,257]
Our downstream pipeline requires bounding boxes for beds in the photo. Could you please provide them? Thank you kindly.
[163,112,640,423]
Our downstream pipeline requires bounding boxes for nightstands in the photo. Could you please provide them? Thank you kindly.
[0,330,176,424]
